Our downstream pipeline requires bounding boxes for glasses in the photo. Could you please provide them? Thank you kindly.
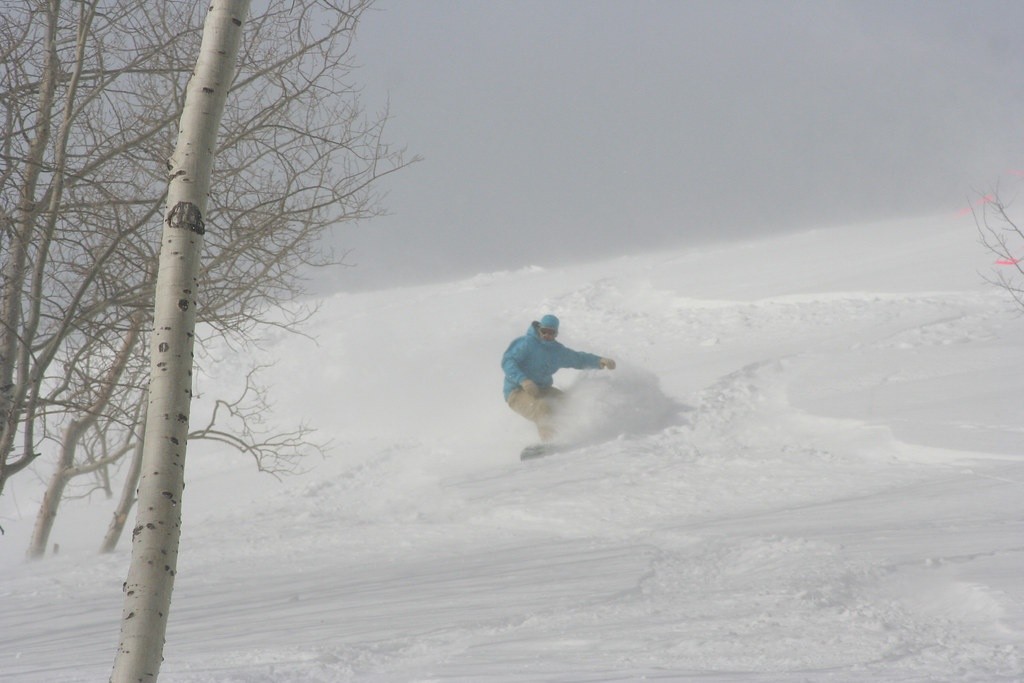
[540,328,556,336]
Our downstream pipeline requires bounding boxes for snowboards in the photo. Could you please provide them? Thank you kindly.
[521,427,619,460]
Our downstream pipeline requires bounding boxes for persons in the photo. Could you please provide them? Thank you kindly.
[501,313,616,444]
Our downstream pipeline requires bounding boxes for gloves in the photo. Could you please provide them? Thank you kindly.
[598,358,615,369]
[521,378,540,397]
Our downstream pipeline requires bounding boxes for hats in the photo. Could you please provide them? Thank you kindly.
[540,315,559,329]
